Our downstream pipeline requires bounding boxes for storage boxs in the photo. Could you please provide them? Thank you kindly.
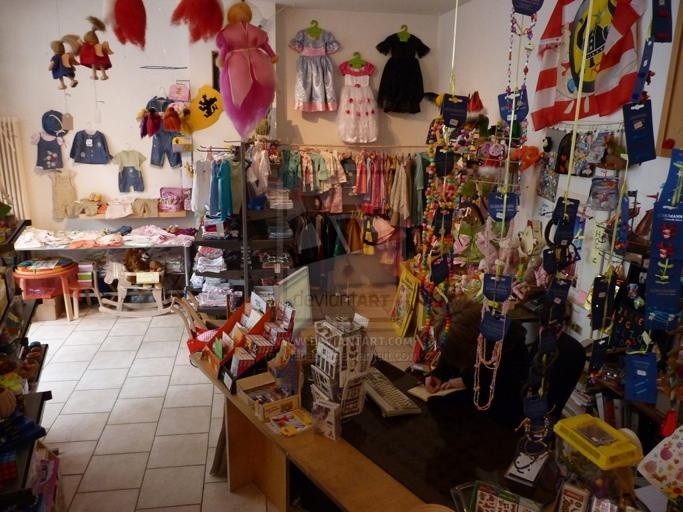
[552,413,639,494]
[32,297,63,321]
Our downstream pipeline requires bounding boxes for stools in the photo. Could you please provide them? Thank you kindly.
[11,256,77,321]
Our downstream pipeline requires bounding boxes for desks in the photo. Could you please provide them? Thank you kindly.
[186,344,522,510]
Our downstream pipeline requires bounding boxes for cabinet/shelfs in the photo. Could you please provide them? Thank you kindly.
[0,297,52,498]
[15,218,291,316]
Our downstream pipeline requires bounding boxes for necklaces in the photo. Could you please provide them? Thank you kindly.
[416,9,537,412]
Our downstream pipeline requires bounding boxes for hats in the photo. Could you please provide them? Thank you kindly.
[42,110,69,137]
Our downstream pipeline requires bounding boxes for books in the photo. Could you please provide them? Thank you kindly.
[267,312,376,440]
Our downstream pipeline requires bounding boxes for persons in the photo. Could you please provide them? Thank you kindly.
[80,15,113,80]
[48,34,84,89]
[215,2,278,140]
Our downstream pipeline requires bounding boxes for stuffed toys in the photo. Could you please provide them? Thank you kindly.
[101,0,146,52]
[123,247,150,286]
[145,261,162,272]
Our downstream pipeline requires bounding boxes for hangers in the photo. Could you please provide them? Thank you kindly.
[304,20,411,68]
